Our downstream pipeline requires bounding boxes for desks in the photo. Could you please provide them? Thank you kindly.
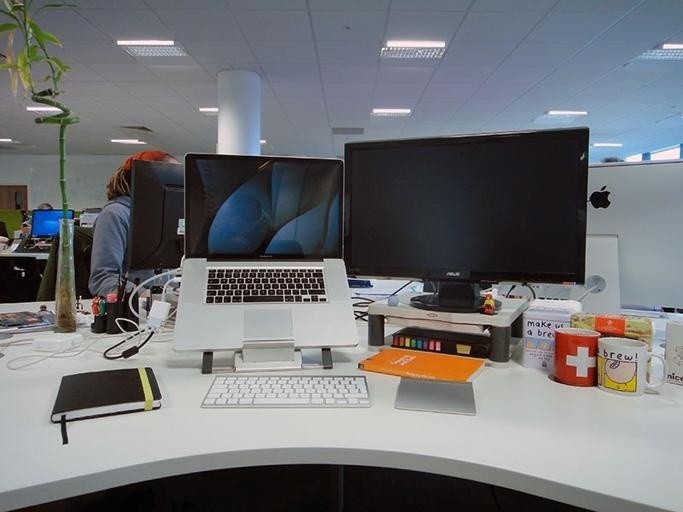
[0,302,683,512]
[0,252,50,261]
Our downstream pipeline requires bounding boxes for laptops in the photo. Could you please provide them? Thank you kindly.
[173,152,358,351]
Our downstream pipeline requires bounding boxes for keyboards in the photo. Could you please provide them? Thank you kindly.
[200,374,371,408]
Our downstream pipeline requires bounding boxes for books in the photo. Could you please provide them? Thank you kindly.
[49,365,162,424]
[356,342,486,386]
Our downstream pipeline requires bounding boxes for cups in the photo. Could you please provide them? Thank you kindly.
[596,337,667,397]
[553,326,601,386]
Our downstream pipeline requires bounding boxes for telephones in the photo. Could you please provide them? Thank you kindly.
[3,237,27,253]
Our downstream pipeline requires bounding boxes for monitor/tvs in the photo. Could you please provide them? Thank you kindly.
[31,210,75,238]
[497,159,683,316]
[125,160,184,272]
[344,126,590,314]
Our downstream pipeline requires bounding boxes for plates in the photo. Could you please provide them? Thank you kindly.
[598,382,650,398]
[545,372,599,391]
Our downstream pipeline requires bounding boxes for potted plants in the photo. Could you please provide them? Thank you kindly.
[0,0,80,331]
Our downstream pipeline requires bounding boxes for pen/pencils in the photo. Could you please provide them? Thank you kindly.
[117,266,140,302]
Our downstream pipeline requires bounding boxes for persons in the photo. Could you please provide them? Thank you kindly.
[18,203,53,234]
[85,149,184,301]
